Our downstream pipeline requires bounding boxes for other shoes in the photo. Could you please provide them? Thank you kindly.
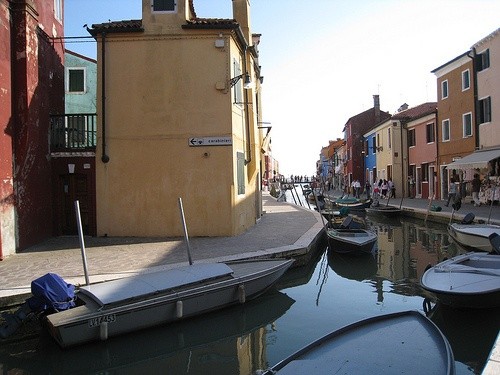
[478,202,483,207]
[473,204,478,207]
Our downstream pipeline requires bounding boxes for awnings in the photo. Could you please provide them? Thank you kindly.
[445,149,500,170]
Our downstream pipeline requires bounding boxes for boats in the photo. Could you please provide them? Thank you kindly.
[418,250,500,313]
[445,167,500,256]
[36,195,297,350]
[294,173,407,254]
[254,309,457,375]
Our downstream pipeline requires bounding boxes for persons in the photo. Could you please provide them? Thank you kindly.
[444,178,456,207]
[463,173,482,208]
[364,180,371,198]
[290,175,308,182]
[325,180,331,192]
[351,179,362,199]
[373,178,396,200]
